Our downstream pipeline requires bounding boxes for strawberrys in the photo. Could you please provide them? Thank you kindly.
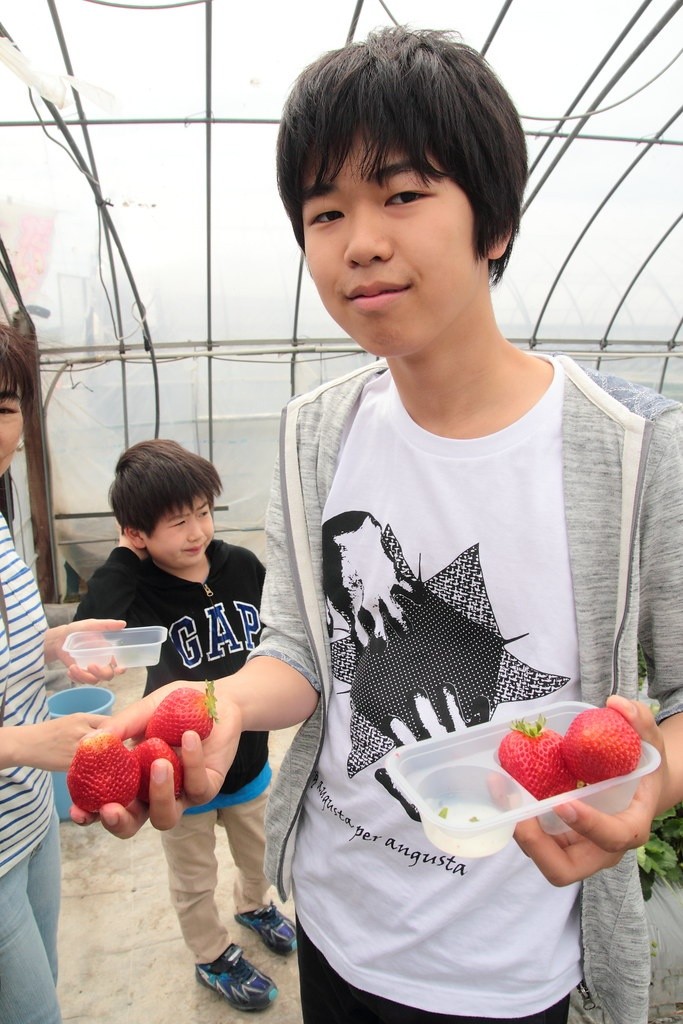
[561,707,641,784]
[66,732,142,813]
[498,727,579,801]
[144,687,214,747]
[127,737,184,804]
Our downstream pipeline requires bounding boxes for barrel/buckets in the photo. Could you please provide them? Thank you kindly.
[44,686,115,823]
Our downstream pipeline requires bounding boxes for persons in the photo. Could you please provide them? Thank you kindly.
[0,324,127,1024]
[70,27,682,1023]
[72,439,296,1012]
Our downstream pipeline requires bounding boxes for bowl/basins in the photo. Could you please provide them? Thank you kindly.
[385,701,662,859]
[61,625,168,670]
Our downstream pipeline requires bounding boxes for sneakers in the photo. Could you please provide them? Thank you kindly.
[195,943,277,1010]
[234,900,296,955]
